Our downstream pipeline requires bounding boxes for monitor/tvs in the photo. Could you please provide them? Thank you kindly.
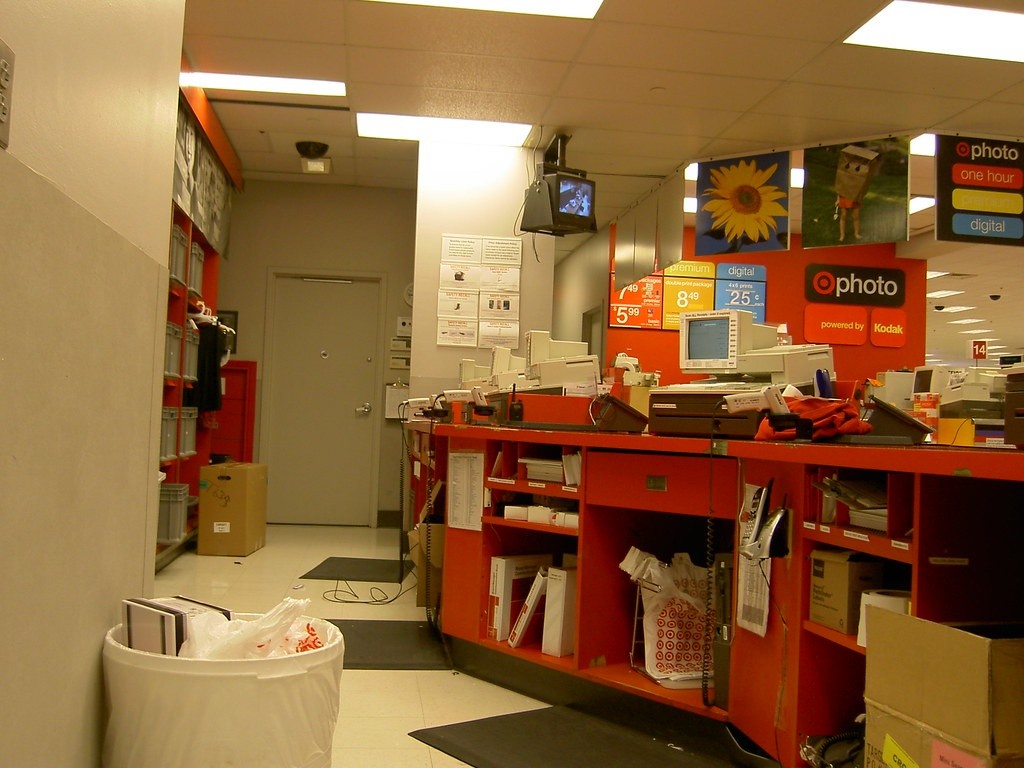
[458,330,588,390]
[679,311,777,374]
[964,367,1006,399]
[911,366,950,401]
[544,172,595,229]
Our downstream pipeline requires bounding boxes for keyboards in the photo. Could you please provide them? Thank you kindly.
[648,383,771,394]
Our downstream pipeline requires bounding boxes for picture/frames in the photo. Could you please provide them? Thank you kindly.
[218,310,238,354]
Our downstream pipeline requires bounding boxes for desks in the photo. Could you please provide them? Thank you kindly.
[395,418,1024,768]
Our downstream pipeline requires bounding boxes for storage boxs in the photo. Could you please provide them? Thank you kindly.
[161,208,205,552]
[406,478,446,608]
[199,460,267,558]
[809,550,885,635]
[856,597,1024,768]
[484,505,581,657]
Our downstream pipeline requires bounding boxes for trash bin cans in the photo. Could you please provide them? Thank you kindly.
[102,611,345,768]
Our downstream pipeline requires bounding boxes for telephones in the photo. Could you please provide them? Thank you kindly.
[738,479,790,559]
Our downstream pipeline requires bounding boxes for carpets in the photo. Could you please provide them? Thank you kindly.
[301,555,416,584]
[409,704,738,768]
[318,617,452,672]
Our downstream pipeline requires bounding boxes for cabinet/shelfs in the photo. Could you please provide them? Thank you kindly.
[156,197,220,574]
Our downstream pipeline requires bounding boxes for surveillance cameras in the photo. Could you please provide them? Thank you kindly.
[935,306,944,310]
[989,295,1001,300]
[296,141,329,159]
